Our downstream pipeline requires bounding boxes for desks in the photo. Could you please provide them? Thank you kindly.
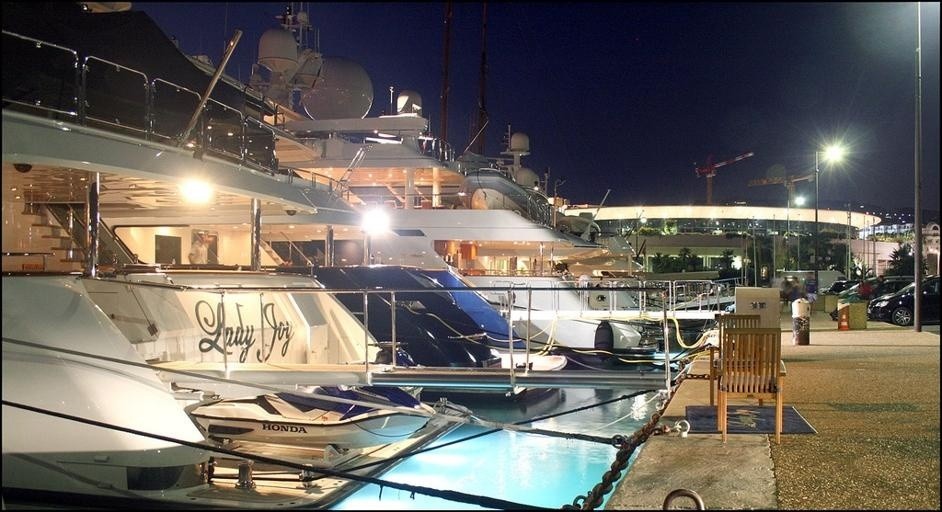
[713,359,787,390]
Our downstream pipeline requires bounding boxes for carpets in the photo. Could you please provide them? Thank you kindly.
[685,405,818,433]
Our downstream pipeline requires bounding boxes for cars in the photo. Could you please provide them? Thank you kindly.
[818,271,940,331]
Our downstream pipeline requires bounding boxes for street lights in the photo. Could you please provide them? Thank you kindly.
[794,195,806,270]
[811,139,850,298]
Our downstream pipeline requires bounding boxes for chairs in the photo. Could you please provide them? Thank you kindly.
[718,328,783,444]
[710,315,764,407]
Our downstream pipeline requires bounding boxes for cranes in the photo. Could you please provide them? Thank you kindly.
[746,171,816,209]
[694,148,754,207]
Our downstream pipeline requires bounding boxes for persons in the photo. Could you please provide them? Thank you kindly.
[802,272,818,315]
[782,275,801,307]
[875,275,884,296]
[187,230,213,264]
